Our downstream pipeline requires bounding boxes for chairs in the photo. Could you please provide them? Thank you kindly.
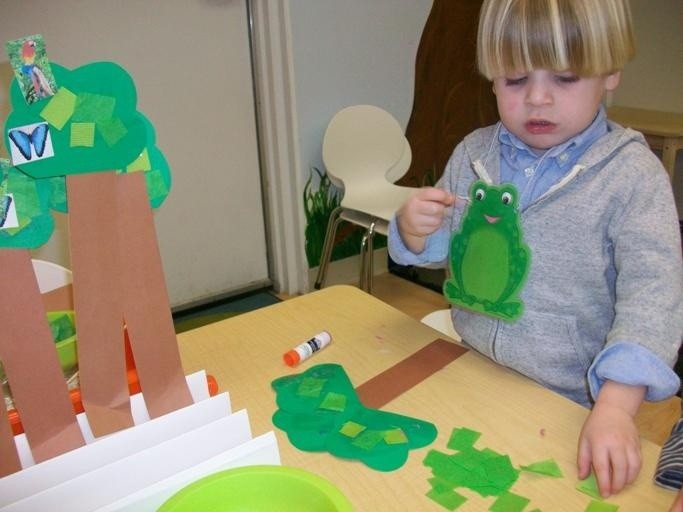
[313,103,425,295]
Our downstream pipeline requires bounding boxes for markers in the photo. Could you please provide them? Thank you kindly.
[283,331,331,367]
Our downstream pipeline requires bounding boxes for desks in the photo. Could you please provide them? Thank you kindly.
[173,283,683,512]
[603,104,683,186]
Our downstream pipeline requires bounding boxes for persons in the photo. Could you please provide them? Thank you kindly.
[647,409,683,511]
[384,1,682,503]
